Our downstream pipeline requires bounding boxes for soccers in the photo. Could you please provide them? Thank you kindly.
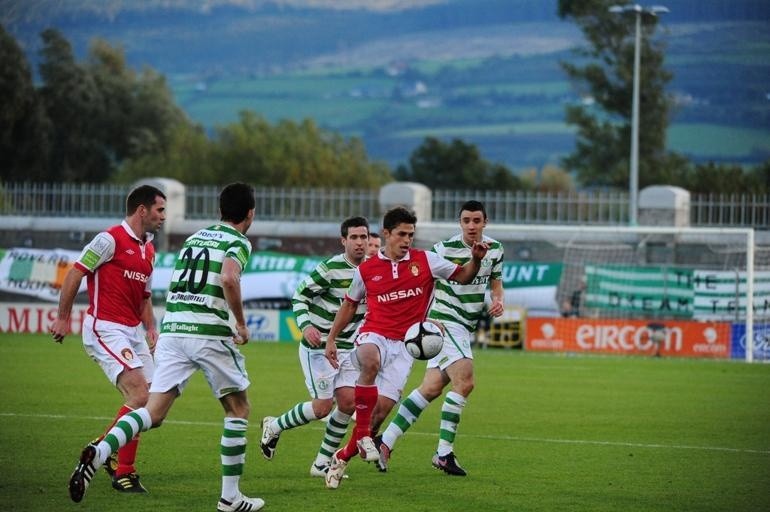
[403,321,444,360]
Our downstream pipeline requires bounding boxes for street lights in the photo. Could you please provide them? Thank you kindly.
[606,2,670,226]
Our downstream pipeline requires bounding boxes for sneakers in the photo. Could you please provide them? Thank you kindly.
[260,416,280,459]
[69,438,148,502]
[310,434,393,489]
[217,493,265,512]
[432,452,466,476]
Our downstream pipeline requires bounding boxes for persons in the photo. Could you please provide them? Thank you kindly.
[261,216,370,479]
[318,228,382,424]
[68,182,265,512]
[562,278,586,319]
[50,184,167,495]
[373,200,505,476]
[475,296,493,351]
[325,207,493,485]
